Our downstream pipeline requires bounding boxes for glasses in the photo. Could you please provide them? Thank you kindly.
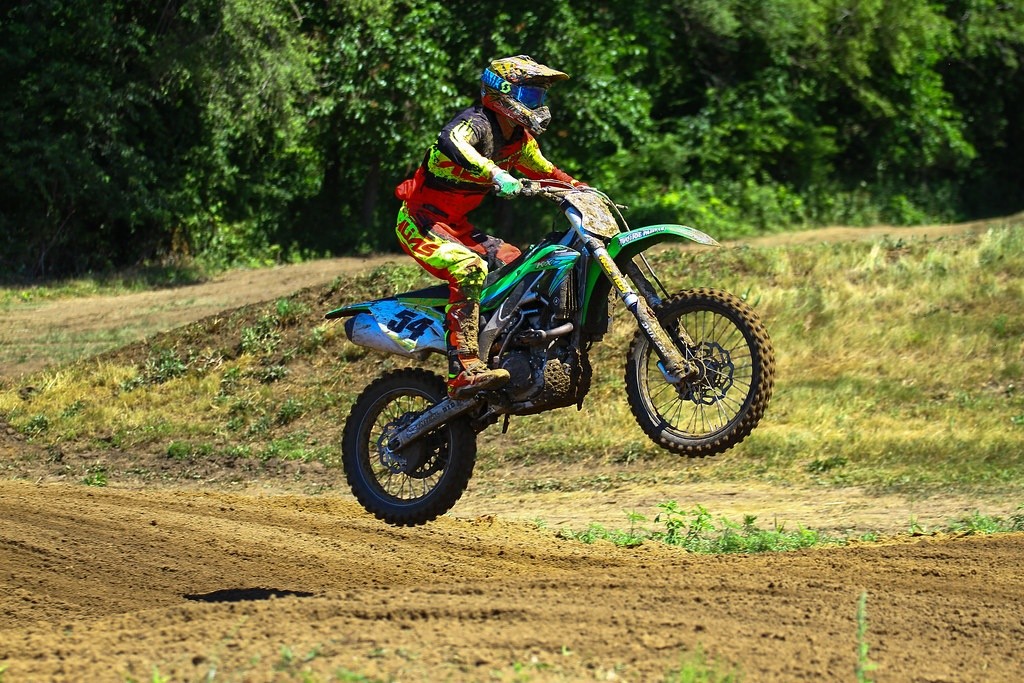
[483,70,547,110]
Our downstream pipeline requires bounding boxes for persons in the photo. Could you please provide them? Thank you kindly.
[395,54,589,401]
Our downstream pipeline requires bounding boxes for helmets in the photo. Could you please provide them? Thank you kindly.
[481,55,570,135]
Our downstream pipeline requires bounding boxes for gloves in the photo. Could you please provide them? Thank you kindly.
[493,170,523,200]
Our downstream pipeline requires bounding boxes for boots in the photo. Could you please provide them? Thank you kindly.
[444,300,510,400]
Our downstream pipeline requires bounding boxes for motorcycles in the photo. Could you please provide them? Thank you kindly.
[323,178,778,528]
